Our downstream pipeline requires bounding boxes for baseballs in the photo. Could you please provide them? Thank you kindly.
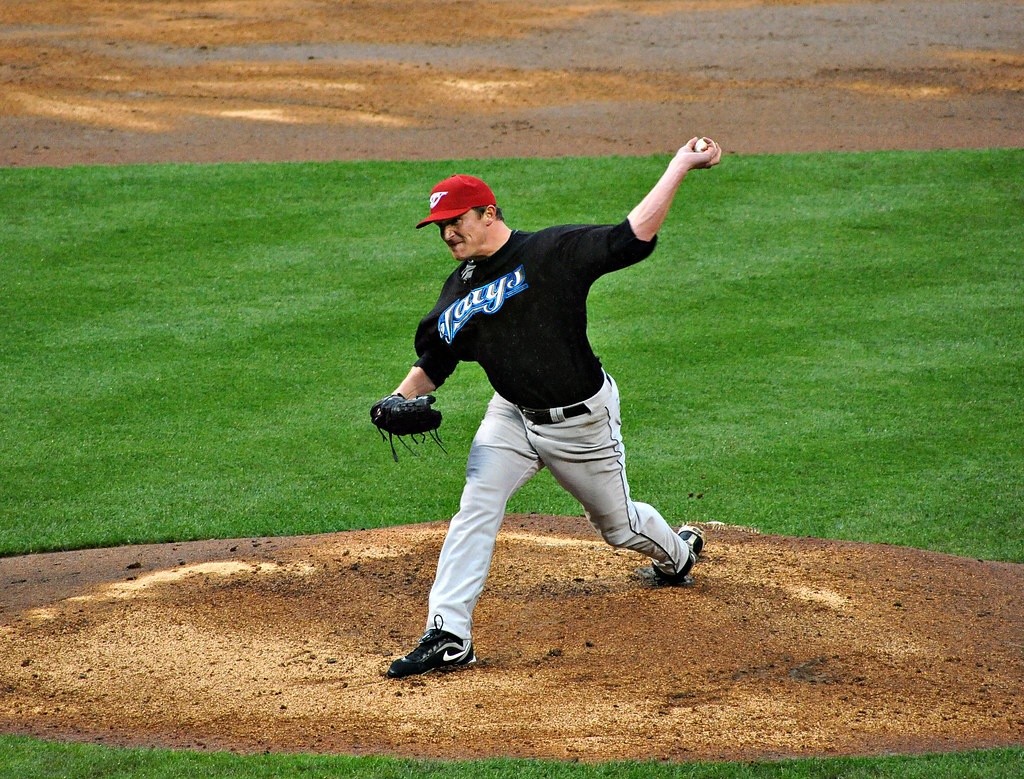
[695,137,708,153]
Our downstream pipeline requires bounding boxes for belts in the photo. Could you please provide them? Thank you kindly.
[518,374,612,425]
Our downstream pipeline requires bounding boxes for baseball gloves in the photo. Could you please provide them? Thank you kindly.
[370,393,442,436]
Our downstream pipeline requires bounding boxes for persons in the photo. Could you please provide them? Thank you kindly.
[370,136,723,678]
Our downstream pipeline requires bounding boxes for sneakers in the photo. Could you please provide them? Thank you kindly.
[653,526,706,583]
[387,614,478,679]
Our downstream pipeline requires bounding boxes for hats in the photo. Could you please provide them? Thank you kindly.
[415,175,496,229]
